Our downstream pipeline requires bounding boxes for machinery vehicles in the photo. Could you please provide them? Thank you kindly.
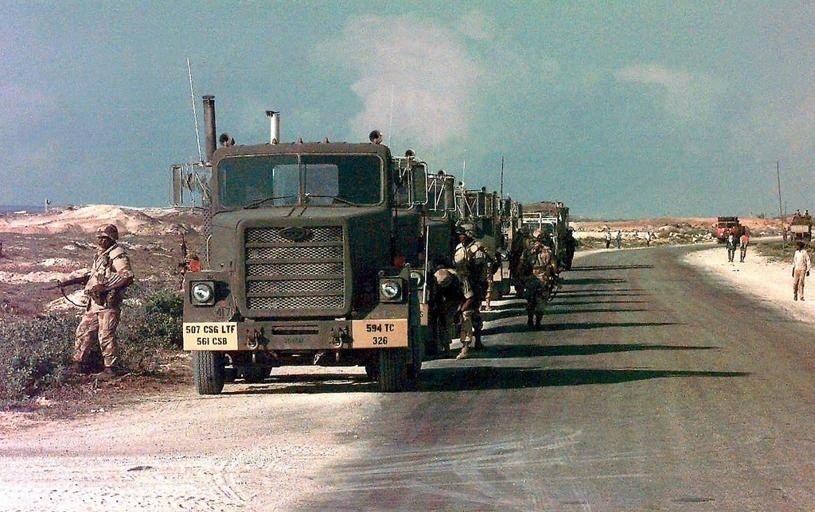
[427,155,574,355]
[170,93,430,393]
[787,215,813,241]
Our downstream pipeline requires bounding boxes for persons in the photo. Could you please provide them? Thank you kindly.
[740,232,749,262]
[511,227,558,330]
[451,223,492,363]
[790,240,813,302]
[605,230,612,249]
[725,232,737,261]
[781,208,811,241]
[616,229,623,249]
[69,224,134,372]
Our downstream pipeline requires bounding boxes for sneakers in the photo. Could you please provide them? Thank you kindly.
[96,367,118,381]
[68,362,91,372]
[456,340,487,360]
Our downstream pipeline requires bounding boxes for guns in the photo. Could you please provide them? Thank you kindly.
[43,276,89,290]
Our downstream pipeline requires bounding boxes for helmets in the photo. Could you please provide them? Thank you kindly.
[456,224,475,239]
[532,229,544,241]
[95,224,120,241]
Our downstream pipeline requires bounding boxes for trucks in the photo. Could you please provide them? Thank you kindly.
[711,215,747,242]
